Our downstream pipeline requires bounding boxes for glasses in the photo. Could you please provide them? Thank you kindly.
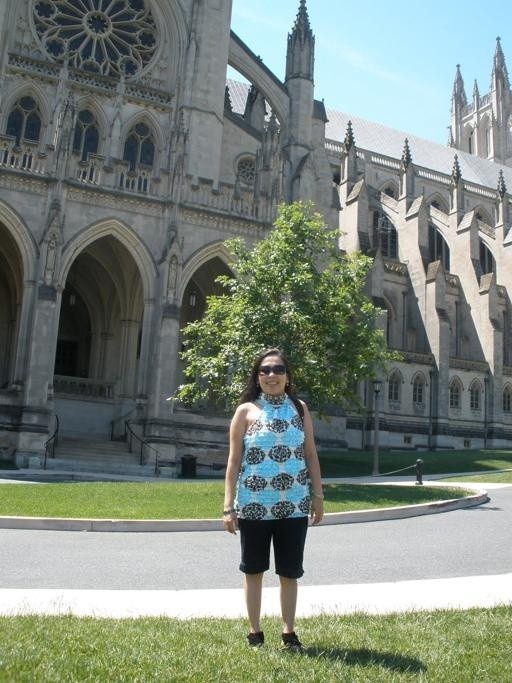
[256,365,287,375]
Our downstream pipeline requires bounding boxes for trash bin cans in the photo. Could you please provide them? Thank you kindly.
[181,454,197,475]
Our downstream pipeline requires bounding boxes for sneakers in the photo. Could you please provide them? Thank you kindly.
[247,639,268,652]
[279,640,310,653]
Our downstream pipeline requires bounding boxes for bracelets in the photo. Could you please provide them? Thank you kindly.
[311,491,323,499]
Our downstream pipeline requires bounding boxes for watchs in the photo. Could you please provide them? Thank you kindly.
[223,509,235,515]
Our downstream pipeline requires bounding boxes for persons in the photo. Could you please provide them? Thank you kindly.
[222,349,323,648]
